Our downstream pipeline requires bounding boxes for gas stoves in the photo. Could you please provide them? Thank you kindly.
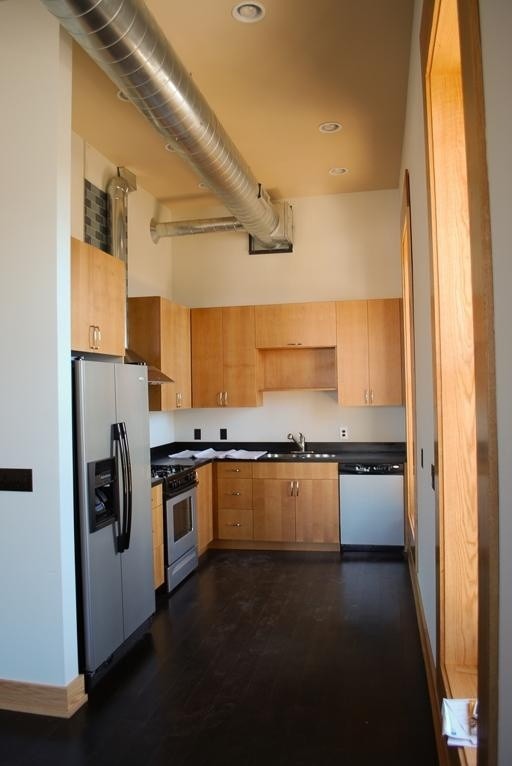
[150,464,199,501]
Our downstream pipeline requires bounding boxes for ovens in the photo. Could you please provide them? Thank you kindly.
[166,486,200,593]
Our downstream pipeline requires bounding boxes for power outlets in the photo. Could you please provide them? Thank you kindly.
[193,428,201,440]
[340,428,347,438]
[219,429,227,440]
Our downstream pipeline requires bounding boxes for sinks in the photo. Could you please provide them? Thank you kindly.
[258,453,336,458]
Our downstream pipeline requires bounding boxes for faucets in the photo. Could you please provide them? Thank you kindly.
[287,432,305,452]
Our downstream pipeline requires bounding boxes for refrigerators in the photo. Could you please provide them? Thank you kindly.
[72,359,159,691]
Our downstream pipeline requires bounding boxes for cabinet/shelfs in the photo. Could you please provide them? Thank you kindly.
[255,301,337,392]
[191,305,263,407]
[151,482,165,591]
[195,463,214,557]
[336,298,405,407]
[253,463,340,551]
[128,296,192,411]
[72,237,126,356]
[217,462,253,549]
